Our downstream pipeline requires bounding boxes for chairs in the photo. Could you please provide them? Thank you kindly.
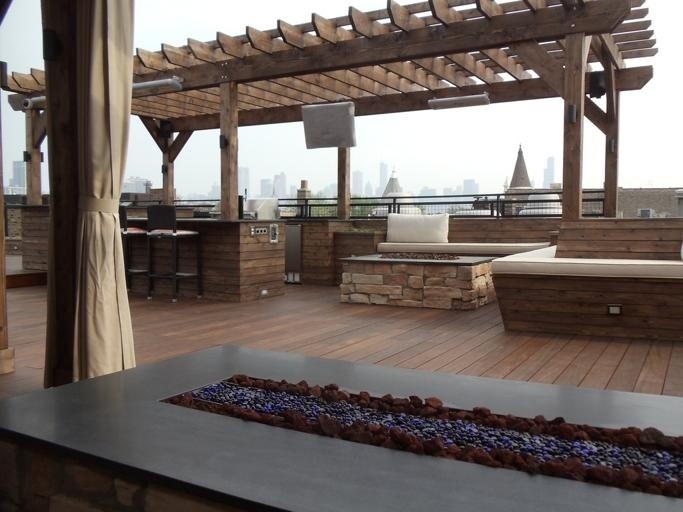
[119,203,204,303]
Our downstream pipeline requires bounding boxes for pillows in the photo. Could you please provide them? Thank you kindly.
[386,212,450,243]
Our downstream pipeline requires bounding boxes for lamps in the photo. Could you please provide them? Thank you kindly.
[608,138,615,153]
[568,103,577,124]
[427,90,491,111]
[30,75,184,109]
[219,134,226,149]
[162,164,167,173]
[23,151,44,162]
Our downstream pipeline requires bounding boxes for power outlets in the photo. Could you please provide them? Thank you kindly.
[256,228,267,235]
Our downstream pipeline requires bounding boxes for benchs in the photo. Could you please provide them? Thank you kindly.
[377,217,683,345]
